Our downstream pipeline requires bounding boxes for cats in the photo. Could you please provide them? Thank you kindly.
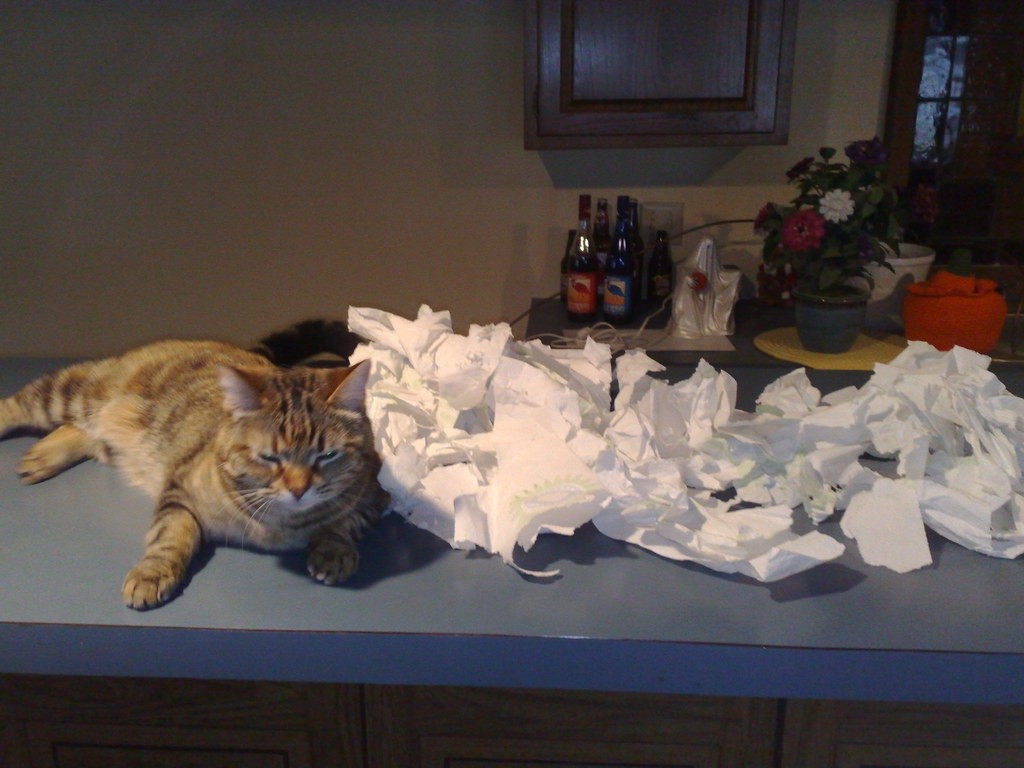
[0,337,392,610]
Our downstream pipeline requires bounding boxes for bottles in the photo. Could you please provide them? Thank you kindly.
[559,229,577,304]
[567,193,598,323]
[629,199,644,306]
[603,195,634,326]
[592,198,613,303]
[647,230,673,307]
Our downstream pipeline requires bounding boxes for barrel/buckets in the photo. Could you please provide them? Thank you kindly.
[862,240,935,331]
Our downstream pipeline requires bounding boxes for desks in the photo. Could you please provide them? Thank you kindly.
[0,356,1024,768]
[523,297,1024,413]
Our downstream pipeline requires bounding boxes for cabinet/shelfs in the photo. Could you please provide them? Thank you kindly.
[522,0,797,150]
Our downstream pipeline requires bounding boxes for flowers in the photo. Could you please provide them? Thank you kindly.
[755,135,901,293]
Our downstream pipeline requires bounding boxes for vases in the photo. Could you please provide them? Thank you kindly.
[795,290,865,351]
[867,239,933,293]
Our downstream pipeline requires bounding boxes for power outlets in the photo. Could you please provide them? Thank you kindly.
[639,204,685,244]
